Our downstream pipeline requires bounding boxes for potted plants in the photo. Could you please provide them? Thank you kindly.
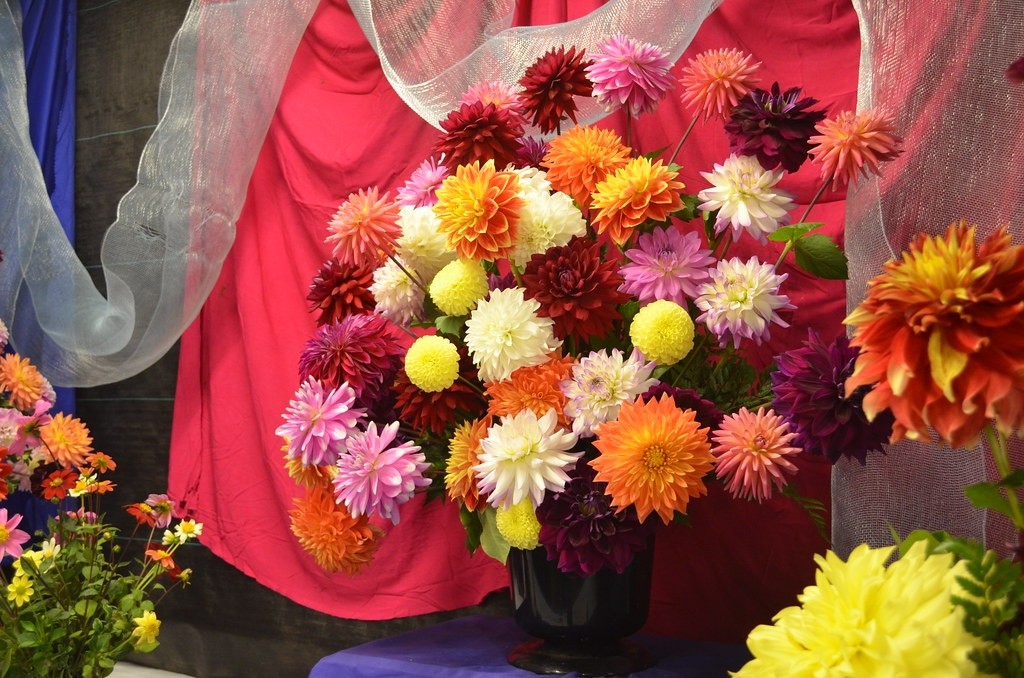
[495,494,656,672]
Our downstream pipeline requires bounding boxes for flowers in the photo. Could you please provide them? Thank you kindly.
[0,312,205,678]
[268,46,1020,676]
